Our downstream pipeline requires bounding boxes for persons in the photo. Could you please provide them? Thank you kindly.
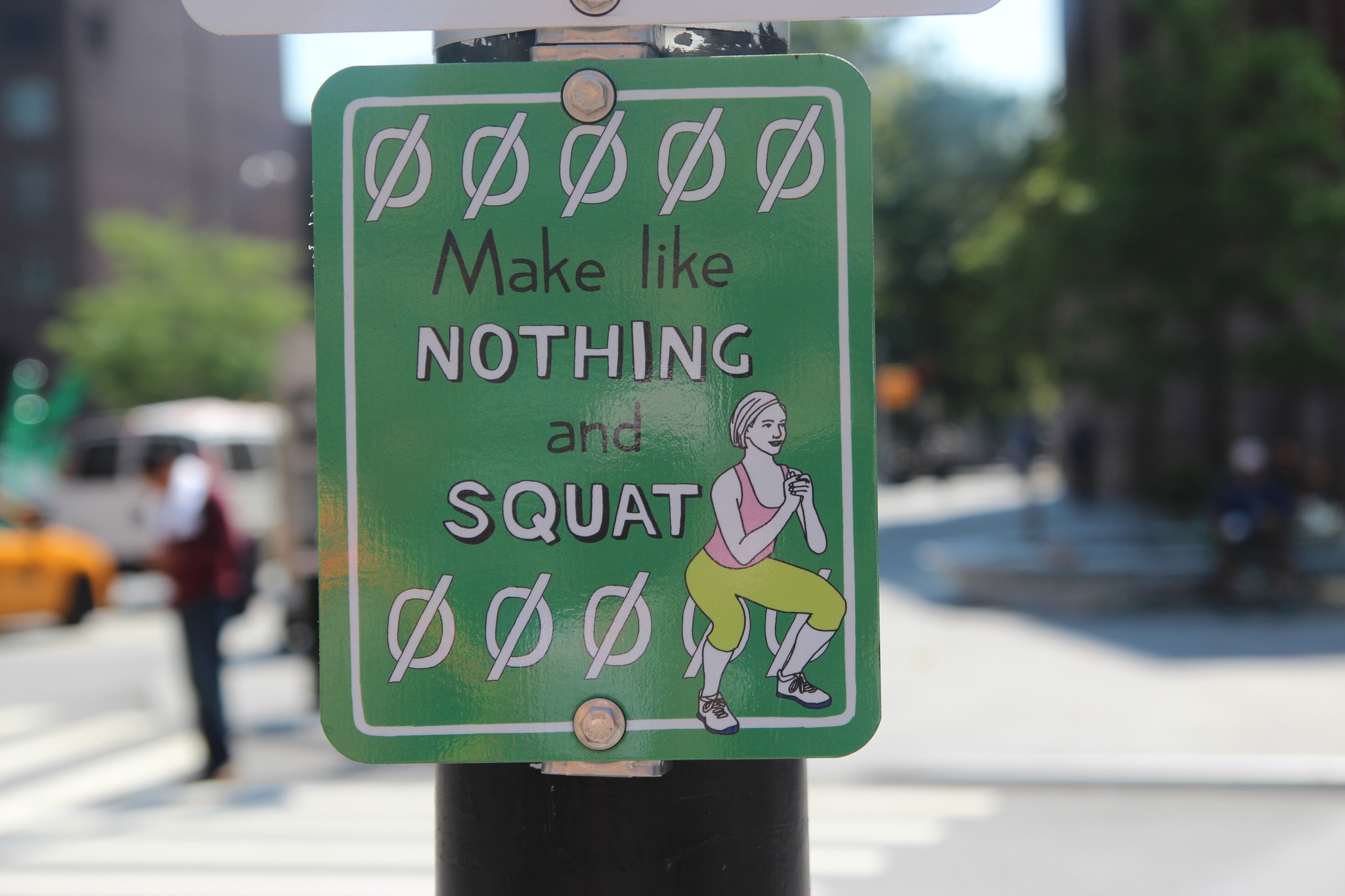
[139,447,242,778]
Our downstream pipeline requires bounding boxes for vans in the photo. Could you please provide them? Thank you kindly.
[43,397,288,573]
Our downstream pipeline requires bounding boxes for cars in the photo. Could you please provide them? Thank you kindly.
[0,487,118,628]
